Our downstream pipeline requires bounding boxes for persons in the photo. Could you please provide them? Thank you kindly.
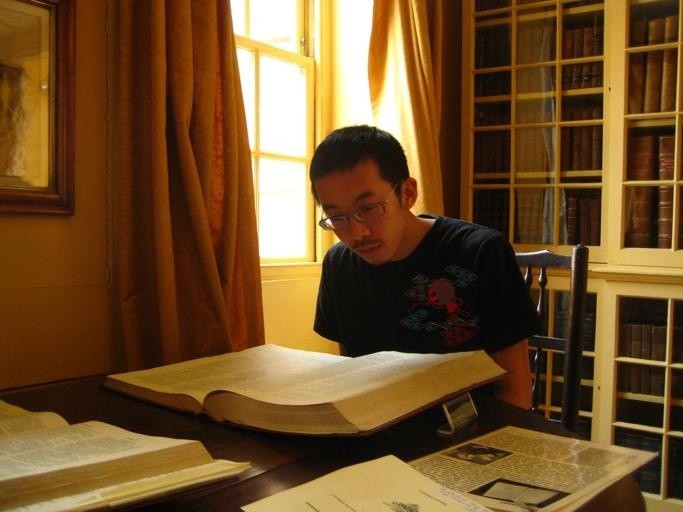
[308,124,537,415]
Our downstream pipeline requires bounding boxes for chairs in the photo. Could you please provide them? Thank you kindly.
[501,245,589,428]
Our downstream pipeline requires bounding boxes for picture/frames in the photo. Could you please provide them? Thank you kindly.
[0,0,76,214]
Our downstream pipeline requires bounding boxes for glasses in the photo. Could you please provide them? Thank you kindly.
[318,184,401,232]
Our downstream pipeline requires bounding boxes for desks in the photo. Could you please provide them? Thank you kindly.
[0,367,649,511]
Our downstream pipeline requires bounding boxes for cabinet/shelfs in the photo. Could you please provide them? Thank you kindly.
[459,0,683,511]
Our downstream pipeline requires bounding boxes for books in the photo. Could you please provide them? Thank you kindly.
[99,343,505,435]
[620,321,667,396]
[624,15,683,248]
[0,394,253,511]
[474,0,602,244]
